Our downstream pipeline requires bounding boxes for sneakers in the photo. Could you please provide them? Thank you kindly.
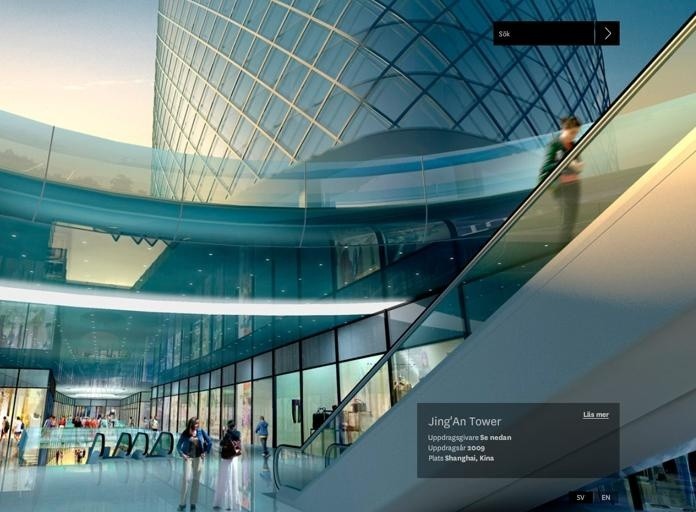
[176,503,196,512]
[260,452,271,458]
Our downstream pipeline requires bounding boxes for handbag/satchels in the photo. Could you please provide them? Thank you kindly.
[230,439,242,456]
[21,422,24,429]
[203,439,209,452]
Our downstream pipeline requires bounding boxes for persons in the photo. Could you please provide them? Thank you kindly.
[74,449,86,464]
[255,416,271,457]
[56,449,63,464]
[176,417,212,512]
[539,116,585,242]
[0,414,159,443]
[213,420,242,511]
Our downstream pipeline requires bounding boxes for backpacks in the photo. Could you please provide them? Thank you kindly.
[4,420,9,433]
